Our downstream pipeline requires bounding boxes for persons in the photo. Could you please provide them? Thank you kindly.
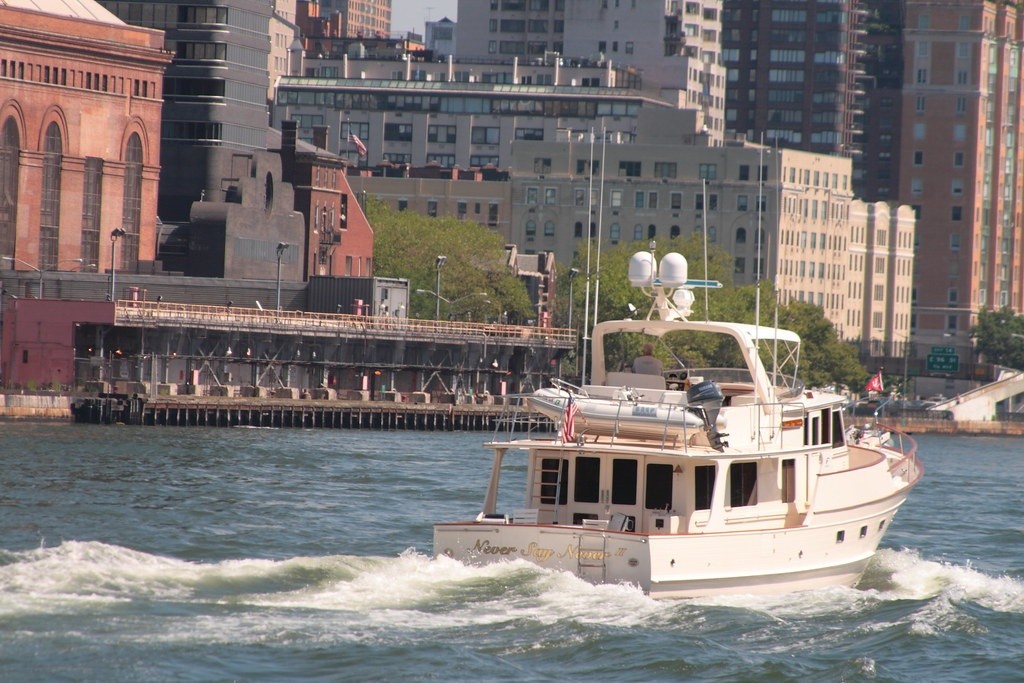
[955,394,960,404]
[632,342,664,377]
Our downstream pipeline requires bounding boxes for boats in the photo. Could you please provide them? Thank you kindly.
[425,239,925,604]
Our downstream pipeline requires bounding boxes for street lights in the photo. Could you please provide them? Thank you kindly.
[109,227,127,301]
[877,329,953,422]
[568,268,580,344]
[273,241,291,315]
[1,255,84,300]
[435,255,448,320]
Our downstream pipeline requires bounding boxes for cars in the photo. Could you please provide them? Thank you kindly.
[843,396,949,418]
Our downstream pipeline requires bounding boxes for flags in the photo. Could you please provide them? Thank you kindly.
[561,395,579,445]
[864,370,884,392]
[347,128,366,156]
